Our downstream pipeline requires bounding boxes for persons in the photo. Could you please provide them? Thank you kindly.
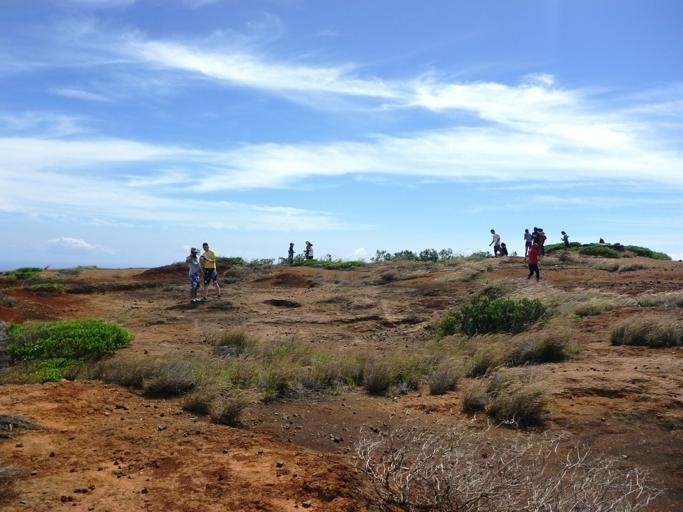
[306,243,313,259]
[559,230,572,250]
[303,240,309,259]
[537,229,546,255]
[530,227,537,241]
[184,247,204,303]
[287,242,296,265]
[523,240,546,282]
[522,228,533,254]
[488,228,501,257]
[198,242,220,300]
[500,241,508,256]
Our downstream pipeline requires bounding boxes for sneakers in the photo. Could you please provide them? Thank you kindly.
[190,294,222,303]
